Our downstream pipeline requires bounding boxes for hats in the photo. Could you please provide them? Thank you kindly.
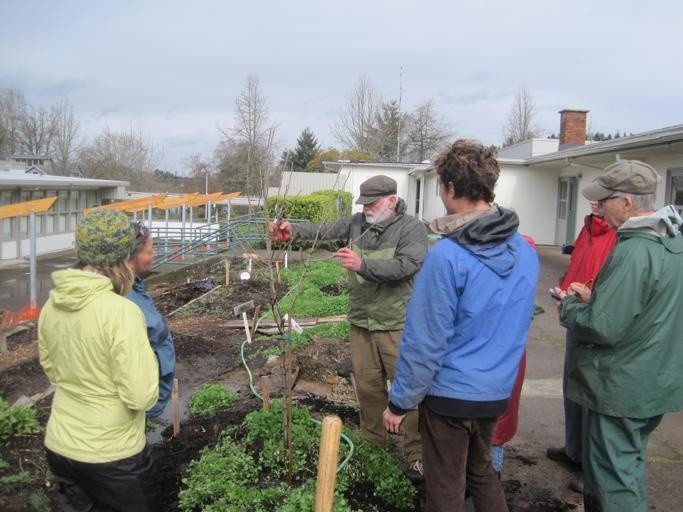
[355,174,398,208]
[74,208,135,267]
[581,159,660,202]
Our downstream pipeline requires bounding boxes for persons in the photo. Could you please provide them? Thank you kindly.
[38,207,168,511]
[546,197,618,495]
[267,175,426,484]
[116,218,177,512]
[546,157,682,512]
[420,234,535,512]
[378,137,540,512]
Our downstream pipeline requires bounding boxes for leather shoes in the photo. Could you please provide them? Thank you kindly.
[547,446,579,466]
[568,474,584,493]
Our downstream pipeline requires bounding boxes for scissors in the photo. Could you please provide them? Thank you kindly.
[273,202,288,242]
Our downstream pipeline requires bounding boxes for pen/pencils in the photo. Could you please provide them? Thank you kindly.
[575,279,594,297]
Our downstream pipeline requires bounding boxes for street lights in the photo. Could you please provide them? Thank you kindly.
[200,166,208,221]
